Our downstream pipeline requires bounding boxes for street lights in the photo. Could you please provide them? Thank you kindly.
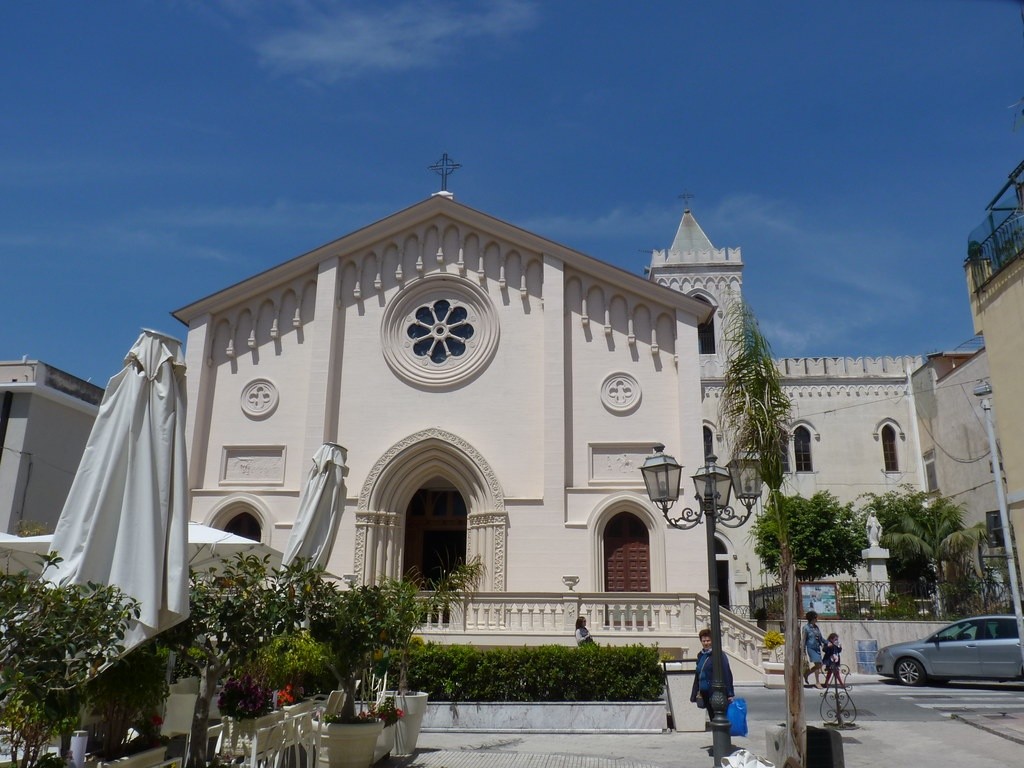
[973,377,1024,685]
[636,441,763,767]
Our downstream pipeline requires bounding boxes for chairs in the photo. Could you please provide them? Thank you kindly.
[232,679,361,768]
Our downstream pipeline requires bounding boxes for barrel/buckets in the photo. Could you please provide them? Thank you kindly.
[855,639,878,674]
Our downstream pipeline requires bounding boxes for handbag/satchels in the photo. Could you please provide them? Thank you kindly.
[696,694,705,708]
[724,697,748,737]
[801,654,810,676]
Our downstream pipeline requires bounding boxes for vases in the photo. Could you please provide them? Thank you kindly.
[85,741,168,768]
[915,599,932,615]
[220,709,284,755]
[370,723,397,765]
[856,598,871,614]
[278,698,314,742]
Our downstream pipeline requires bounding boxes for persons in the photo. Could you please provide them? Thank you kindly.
[575,616,593,648]
[690,629,735,722]
[801,611,828,689]
[823,633,846,688]
[866,510,883,549]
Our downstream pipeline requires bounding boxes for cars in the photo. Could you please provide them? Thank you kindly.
[875,614,1024,687]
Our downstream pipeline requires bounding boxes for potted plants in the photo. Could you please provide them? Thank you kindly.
[765,595,783,620]
[838,580,855,603]
[311,549,487,768]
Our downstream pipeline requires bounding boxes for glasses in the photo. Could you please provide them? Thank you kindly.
[583,620,586,622]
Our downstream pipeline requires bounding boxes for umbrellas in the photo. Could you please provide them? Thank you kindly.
[275,442,349,628]
[0,327,190,729]
[0,519,285,583]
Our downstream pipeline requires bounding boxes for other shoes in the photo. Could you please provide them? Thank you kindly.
[803,673,809,685]
[814,684,823,689]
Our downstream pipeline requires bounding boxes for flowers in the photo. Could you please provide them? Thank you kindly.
[218,675,274,722]
[84,639,171,768]
[273,684,294,709]
[358,692,406,727]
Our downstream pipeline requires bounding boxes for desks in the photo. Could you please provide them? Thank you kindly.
[215,698,314,757]
